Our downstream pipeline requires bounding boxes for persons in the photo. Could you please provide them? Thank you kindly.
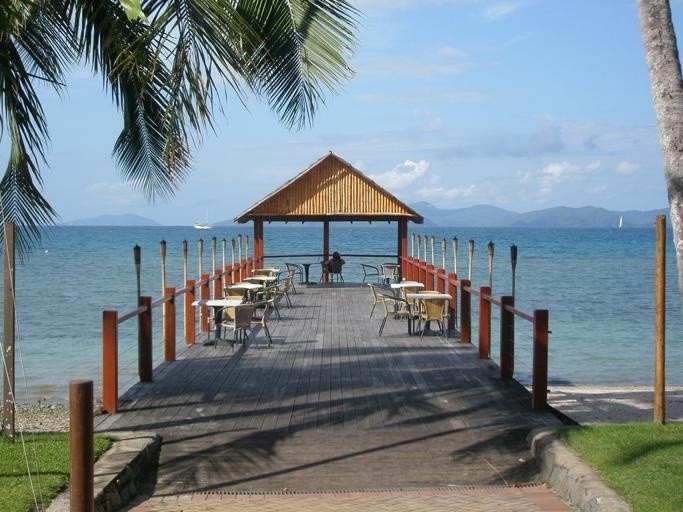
[321,250,344,273]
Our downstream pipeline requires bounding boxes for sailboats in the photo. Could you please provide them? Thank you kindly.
[619,217,623,229]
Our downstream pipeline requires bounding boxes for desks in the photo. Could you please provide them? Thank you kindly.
[190,299,242,345]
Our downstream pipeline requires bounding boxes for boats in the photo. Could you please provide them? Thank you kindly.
[194,224,212,229]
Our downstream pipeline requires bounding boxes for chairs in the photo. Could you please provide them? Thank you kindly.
[212,262,303,353]
[328,261,344,284]
[360,263,452,341]
